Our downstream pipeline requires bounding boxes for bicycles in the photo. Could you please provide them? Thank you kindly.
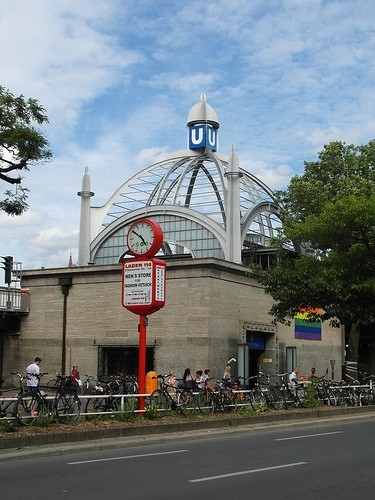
[0,370,375,433]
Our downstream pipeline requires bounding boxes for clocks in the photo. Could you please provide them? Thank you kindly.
[127,218,163,258]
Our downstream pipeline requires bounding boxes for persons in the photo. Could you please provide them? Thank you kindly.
[26,357,41,416]
[167,371,177,392]
[183,368,211,389]
[71,366,79,379]
[223,366,231,382]
[290,367,299,385]
[311,368,319,382]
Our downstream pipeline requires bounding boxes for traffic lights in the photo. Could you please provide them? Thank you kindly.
[1,256,12,273]
